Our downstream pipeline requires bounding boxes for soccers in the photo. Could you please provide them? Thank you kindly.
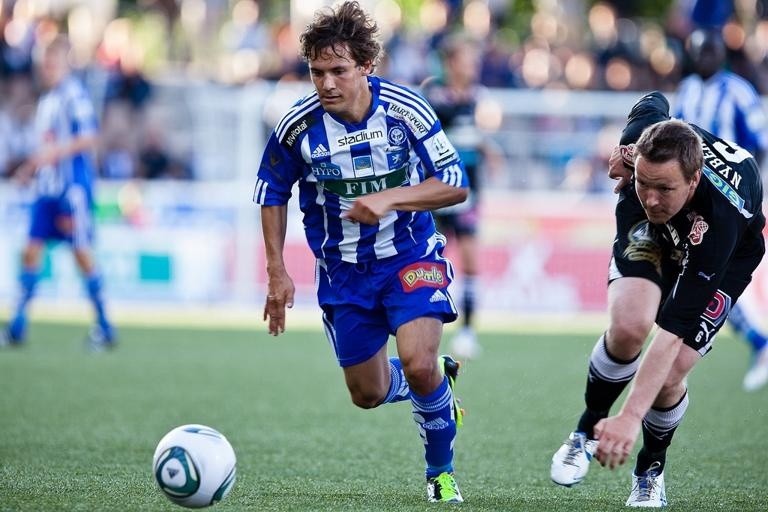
[152,425,237,508]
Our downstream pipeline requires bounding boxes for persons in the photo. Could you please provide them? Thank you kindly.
[544,89,768,508]
[249,0,476,505]
[1,0,766,185]
[2,33,119,352]
[658,26,768,394]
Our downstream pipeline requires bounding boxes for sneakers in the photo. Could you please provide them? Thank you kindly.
[550,432,598,486]
[627,467,666,508]
[438,355,464,430]
[742,342,768,389]
[427,472,463,504]
